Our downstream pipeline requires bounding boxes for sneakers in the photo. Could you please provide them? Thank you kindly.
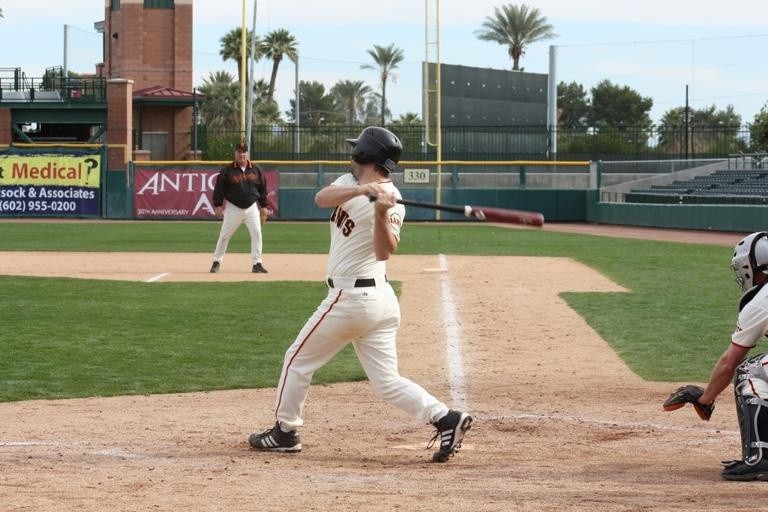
[426,409,474,463]
[210,261,220,273]
[720,453,768,483]
[252,262,268,274]
[248,420,303,454]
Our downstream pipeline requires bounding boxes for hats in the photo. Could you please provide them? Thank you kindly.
[235,143,249,152]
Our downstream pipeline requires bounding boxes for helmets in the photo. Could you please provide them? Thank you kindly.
[730,231,768,292]
[345,126,404,174]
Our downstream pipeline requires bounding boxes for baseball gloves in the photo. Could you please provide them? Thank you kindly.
[663,385,714,421]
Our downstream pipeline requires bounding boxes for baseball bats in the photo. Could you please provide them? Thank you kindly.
[370,192,544,227]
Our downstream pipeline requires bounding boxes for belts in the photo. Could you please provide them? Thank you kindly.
[325,274,388,287]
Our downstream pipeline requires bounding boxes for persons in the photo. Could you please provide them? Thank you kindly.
[249,126,472,462]
[210,142,269,273]
[663,231,768,481]
